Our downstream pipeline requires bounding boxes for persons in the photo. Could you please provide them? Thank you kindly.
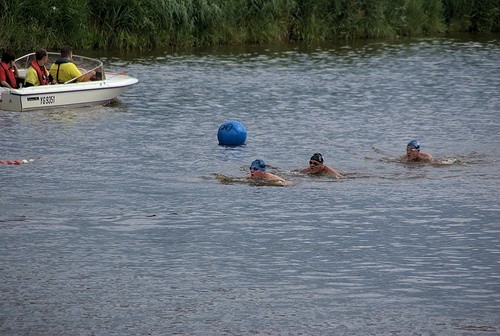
[0,52,19,88]
[49,46,96,84]
[299,152,340,177]
[245,159,283,181]
[401,139,433,162]
[24,48,57,87]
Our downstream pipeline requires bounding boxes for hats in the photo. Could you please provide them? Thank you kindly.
[310,153,324,163]
[407,139,420,151]
[251,159,266,170]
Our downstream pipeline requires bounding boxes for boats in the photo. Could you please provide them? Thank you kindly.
[0,53,139,113]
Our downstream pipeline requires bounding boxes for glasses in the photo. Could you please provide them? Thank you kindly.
[406,148,419,152]
[309,160,319,165]
[249,166,258,170]
